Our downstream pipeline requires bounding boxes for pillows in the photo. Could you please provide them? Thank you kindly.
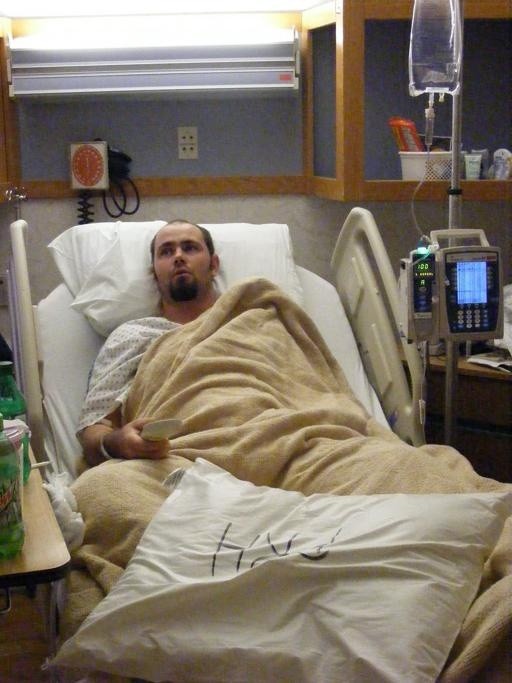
[47,222,303,337]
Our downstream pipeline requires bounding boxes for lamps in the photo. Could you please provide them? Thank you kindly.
[2,24,301,100]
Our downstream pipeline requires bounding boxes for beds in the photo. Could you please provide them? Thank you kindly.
[7,206,511,682]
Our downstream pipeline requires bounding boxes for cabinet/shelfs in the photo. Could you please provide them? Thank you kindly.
[298,0,511,202]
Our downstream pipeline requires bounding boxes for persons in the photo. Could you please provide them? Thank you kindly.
[73,217,221,466]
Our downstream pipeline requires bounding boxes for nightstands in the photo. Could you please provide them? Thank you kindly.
[399,340,512,481]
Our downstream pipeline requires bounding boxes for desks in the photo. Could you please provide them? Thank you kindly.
[1,420,73,662]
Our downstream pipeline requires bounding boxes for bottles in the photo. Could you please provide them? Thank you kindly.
[0,360,31,486]
[0,414,25,560]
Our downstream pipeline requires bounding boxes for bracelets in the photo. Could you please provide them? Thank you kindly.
[99,435,114,460]
